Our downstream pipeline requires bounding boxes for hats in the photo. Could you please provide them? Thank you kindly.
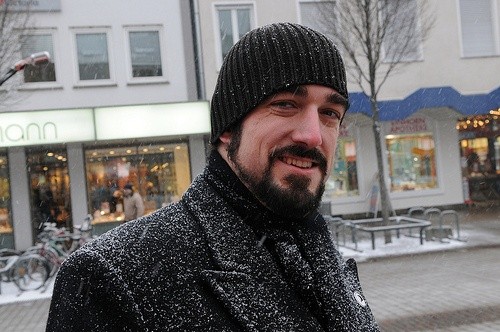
[209,22,348,145]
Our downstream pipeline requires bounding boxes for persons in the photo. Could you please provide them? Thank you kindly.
[32,173,147,229]
[466,151,497,200]
[44,21,381,332]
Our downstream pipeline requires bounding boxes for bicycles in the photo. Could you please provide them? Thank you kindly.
[0,220,90,293]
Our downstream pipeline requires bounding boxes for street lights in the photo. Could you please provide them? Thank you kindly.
[0,51,50,86]
